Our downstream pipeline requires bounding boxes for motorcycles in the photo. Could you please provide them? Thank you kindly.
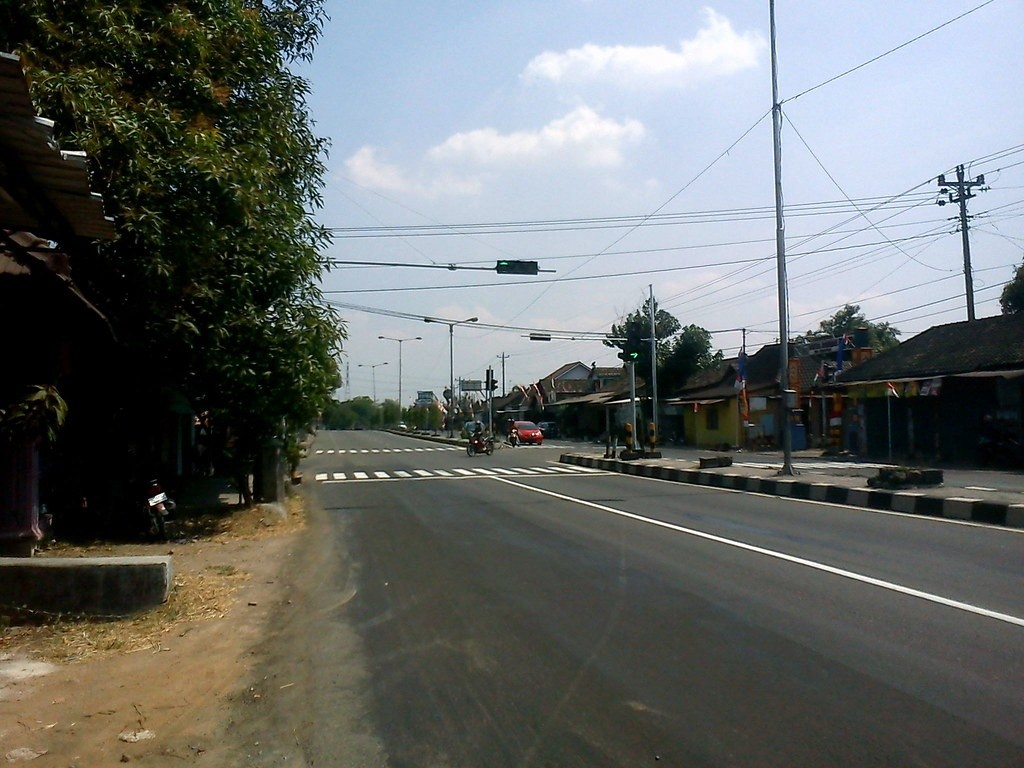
[466,430,494,457]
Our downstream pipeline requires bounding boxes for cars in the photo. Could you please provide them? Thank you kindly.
[460,420,485,438]
[514,421,543,445]
[536,421,559,437]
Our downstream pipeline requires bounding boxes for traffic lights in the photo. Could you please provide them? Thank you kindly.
[624,321,643,362]
[496,260,538,275]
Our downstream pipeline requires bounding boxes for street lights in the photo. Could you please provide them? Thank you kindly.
[378,336,423,423]
[358,362,388,409]
[423,316,478,438]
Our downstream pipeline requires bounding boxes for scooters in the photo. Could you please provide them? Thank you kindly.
[145,476,178,542]
[510,429,518,447]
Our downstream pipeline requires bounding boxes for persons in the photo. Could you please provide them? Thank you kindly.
[470,421,487,451]
